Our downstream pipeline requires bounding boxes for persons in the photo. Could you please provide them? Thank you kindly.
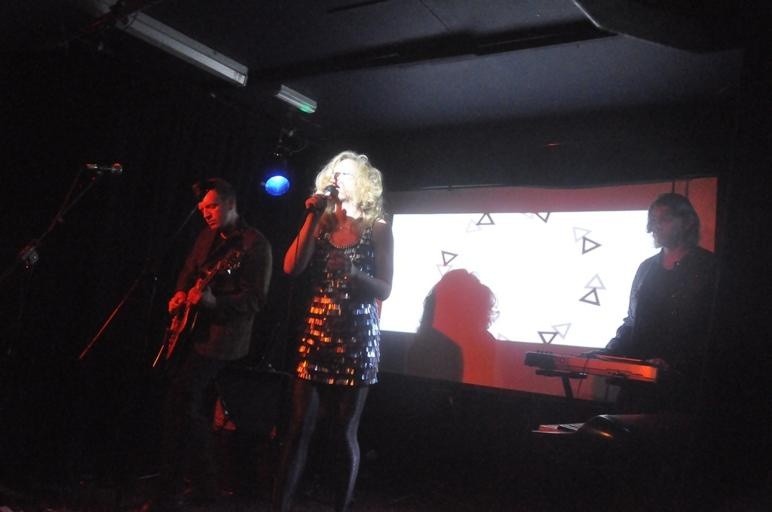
[279,151,394,512]
[605,192,716,415]
[135,178,272,511]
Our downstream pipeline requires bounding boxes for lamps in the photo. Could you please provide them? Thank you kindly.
[273,84,319,115]
[121,13,249,88]
[257,152,294,201]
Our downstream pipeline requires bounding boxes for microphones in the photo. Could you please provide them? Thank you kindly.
[85,163,123,175]
[308,185,336,212]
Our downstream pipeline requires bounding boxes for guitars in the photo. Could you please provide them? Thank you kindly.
[160,248,242,360]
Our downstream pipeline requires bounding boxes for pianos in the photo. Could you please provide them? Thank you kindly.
[524,351,671,384]
